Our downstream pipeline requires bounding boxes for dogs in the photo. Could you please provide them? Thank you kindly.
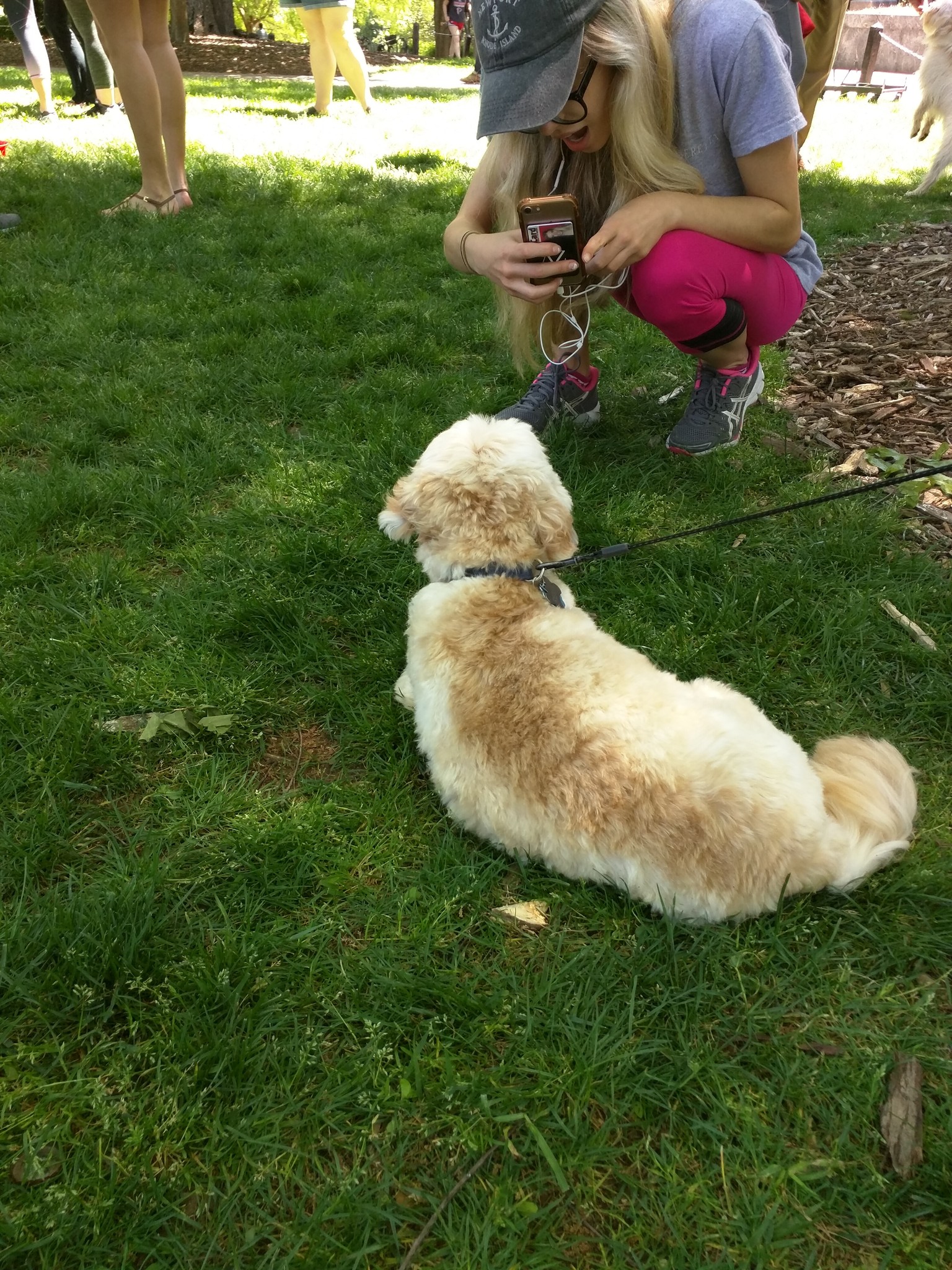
[902,1,952,196]
[378,410,920,927]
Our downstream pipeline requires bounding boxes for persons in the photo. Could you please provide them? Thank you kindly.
[443,0,823,456]
[256,23,267,38]
[63,0,126,118]
[542,223,574,240]
[280,0,376,117]
[86,0,194,216]
[3,0,59,121]
[442,0,472,58]
[756,0,935,174]
[43,0,98,106]
[459,52,482,85]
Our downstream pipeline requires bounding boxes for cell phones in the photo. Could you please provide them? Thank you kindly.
[517,192,587,289]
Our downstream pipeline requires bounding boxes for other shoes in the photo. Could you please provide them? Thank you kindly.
[41,110,56,124]
[63,101,88,116]
[298,107,320,117]
[0,213,21,232]
[84,103,122,117]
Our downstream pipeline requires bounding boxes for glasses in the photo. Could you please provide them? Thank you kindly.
[518,53,598,134]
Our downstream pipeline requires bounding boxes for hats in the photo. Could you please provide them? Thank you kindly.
[471,0,603,140]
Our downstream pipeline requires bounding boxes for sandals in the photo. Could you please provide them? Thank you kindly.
[99,185,190,217]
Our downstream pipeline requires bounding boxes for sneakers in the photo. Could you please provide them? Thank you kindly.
[662,341,766,456]
[492,350,603,438]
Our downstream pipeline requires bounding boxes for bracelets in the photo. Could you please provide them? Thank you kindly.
[460,231,482,276]
